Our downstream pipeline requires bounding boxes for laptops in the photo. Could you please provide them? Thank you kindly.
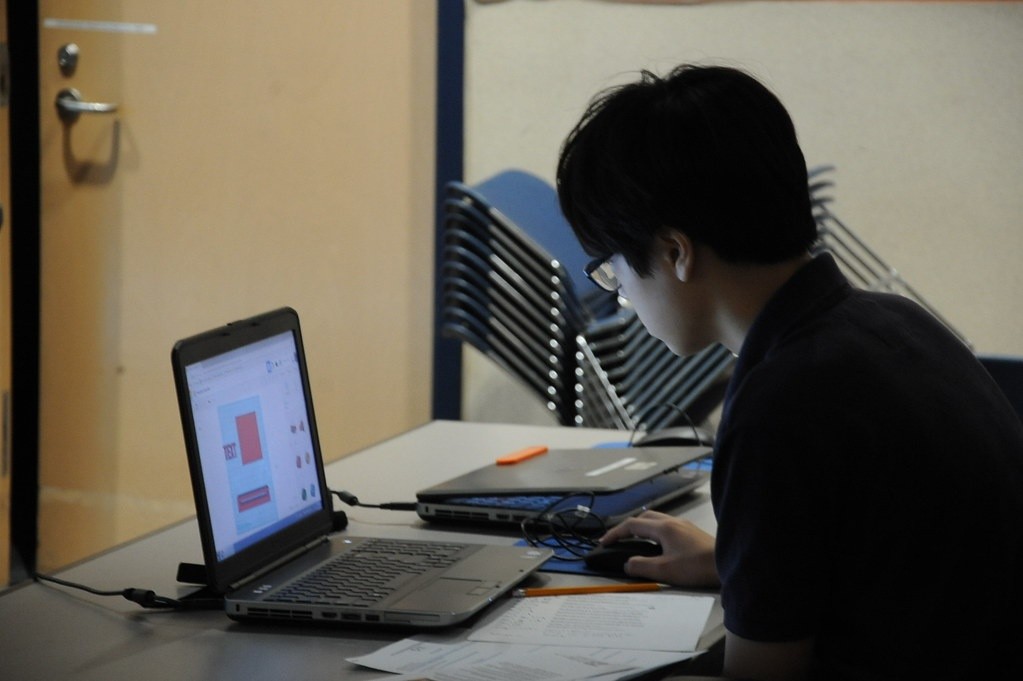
[415,445,712,535]
[172,305,556,627]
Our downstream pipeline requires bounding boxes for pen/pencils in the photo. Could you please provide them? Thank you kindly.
[506,583,672,599]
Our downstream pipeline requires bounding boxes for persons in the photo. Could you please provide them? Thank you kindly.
[555,64,1023,681]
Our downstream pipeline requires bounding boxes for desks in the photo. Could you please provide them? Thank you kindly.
[2,418,726,679]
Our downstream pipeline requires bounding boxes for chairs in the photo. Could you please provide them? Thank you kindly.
[441,162,983,427]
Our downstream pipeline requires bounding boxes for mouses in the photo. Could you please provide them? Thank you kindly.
[580,540,664,574]
[631,427,715,447]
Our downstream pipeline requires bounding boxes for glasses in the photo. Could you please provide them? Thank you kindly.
[582,247,621,292]
